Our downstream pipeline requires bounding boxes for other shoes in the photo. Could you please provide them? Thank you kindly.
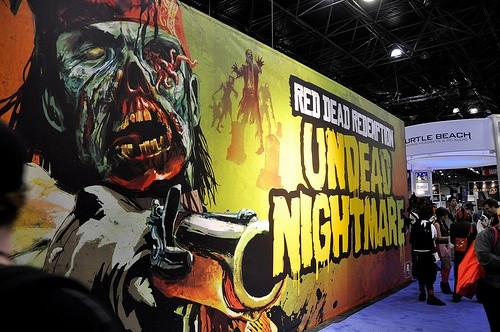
[419,293,426,302]
[427,294,446,306]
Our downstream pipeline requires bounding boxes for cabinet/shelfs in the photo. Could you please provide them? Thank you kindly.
[432,183,441,208]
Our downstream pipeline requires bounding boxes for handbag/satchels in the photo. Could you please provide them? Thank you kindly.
[456,228,498,293]
[454,238,467,251]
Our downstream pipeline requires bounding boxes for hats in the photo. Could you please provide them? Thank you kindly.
[497,207,500,215]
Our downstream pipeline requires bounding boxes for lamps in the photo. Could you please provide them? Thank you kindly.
[391,49,404,59]
[470,108,478,115]
[452,108,461,114]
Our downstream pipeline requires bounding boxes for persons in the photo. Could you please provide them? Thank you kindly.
[450,206,478,303]
[474,206,500,332]
[408,204,446,307]
[409,192,500,267]
[433,207,454,294]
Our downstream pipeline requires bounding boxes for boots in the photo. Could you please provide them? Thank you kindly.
[440,282,454,294]
